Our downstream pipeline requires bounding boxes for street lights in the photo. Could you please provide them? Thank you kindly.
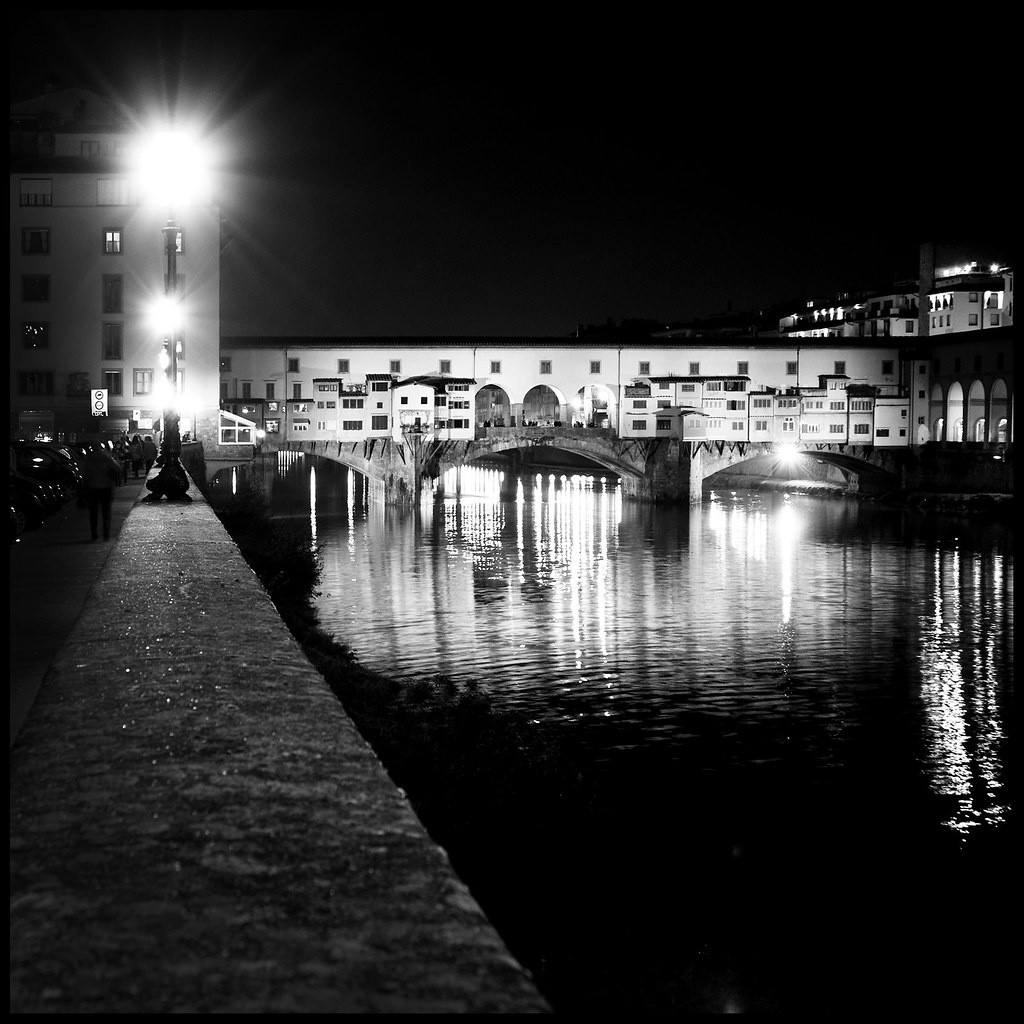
[126,115,218,496]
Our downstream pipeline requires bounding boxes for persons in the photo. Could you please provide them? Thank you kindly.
[112,435,158,487]
[82,442,121,542]
